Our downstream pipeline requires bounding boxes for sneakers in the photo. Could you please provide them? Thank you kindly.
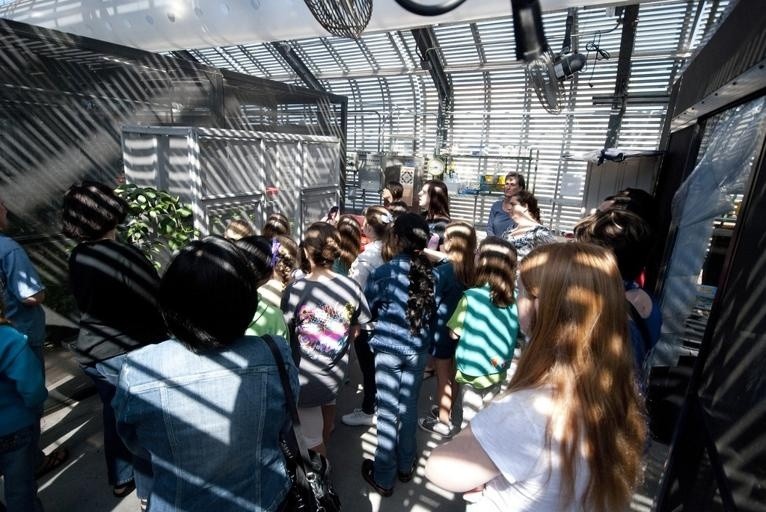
[431,405,454,431]
[342,408,377,425]
[418,416,452,438]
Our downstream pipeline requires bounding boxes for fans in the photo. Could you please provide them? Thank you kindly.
[527,41,585,113]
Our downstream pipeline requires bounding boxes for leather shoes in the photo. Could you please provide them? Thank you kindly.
[398,452,418,482]
[362,459,392,497]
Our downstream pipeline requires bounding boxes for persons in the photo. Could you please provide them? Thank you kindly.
[223,172,559,496]
[110,235,311,511]
[0,203,47,383]
[0,291,48,511]
[425,244,651,511]
[573,188,662,391]
[61,181,161,497]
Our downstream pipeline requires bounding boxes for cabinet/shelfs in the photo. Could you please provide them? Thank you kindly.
[432,145,539,244]
[547,140,588,244]
[121,126,342,282]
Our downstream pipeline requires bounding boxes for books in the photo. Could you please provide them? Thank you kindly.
[676,283,718,352]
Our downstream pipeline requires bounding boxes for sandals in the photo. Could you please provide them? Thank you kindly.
[114,478,148,512]
[35,450,69,479]
[423,370,435,380]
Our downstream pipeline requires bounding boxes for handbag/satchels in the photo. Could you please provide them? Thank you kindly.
[275,449,344,512]
